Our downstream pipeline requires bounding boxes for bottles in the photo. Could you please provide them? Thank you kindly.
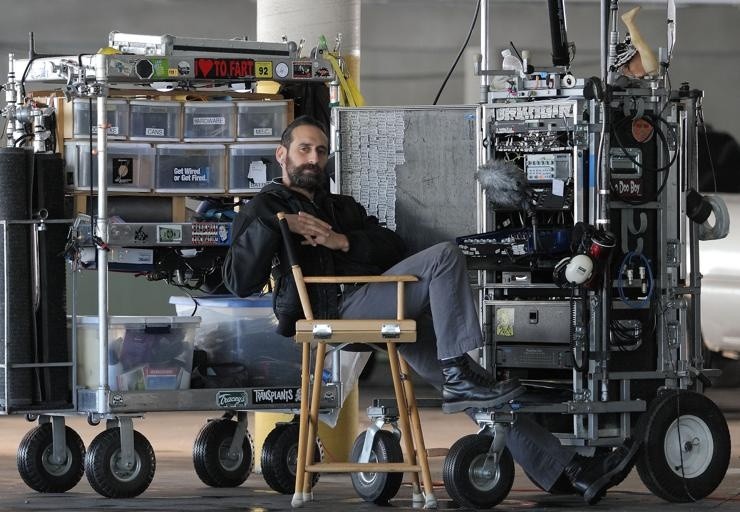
[501,49,523,72]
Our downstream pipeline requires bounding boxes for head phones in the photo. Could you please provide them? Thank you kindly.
[551,255,594,291]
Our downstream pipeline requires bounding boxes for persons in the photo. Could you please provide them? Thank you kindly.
[221,115,644,505]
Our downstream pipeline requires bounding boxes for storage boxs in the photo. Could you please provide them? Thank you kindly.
[64,293,316,399]
[61,99,292,203]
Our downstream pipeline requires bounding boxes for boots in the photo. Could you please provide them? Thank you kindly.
[439,356,524,415]
[566,435,639,503]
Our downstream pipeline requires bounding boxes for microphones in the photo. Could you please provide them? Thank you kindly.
[474,159,538,251]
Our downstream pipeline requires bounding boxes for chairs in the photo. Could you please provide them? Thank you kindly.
[266,210,439,512]
